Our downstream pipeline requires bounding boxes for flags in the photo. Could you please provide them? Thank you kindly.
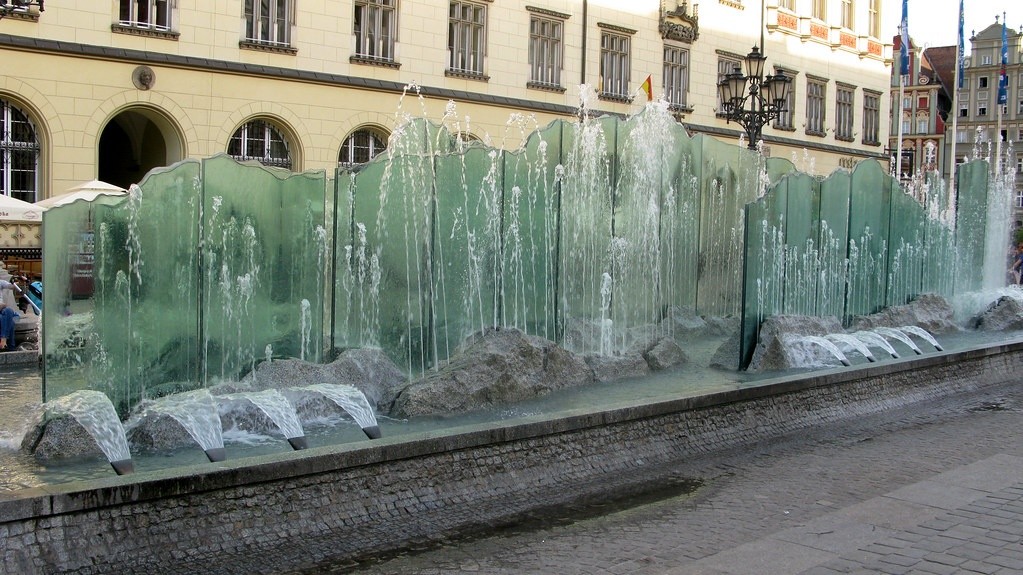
[996,16,1007,104]
[641,76,653,102]
[957,0,964,88]
[898,0,910,76]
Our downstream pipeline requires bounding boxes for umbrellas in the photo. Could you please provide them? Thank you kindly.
[0,193,53,223]
[31,177,133,265]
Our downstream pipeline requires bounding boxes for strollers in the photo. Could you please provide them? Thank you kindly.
[10,274,86,373]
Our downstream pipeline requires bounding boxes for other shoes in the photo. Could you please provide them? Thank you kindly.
[0,344,16,352]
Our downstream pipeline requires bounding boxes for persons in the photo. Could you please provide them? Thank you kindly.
[0,276,27,352]
[1005,242,1023,285]
